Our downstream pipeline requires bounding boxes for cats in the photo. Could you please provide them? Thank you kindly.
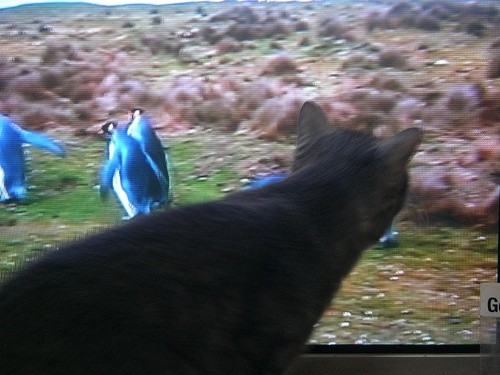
[3,98,425,373]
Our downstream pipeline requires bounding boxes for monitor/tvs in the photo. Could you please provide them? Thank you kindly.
[0,0,500,375]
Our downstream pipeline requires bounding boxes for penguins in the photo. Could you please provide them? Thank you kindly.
[0,108,173,222]
